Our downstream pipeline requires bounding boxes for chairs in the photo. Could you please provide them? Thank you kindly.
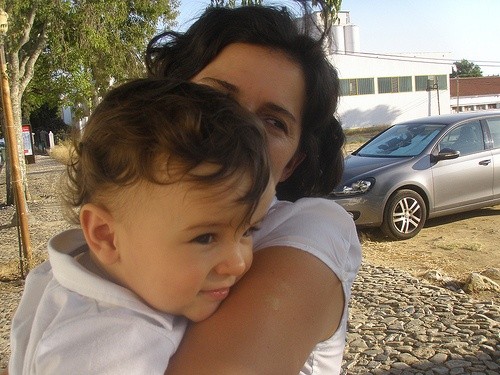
[452,126,478,155]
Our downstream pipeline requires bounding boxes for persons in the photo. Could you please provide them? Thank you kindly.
[138,2,362,375]
[448,126,482,157]
[4,74,278,375]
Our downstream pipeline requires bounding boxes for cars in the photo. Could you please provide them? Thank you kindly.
[318,108,500,240]
